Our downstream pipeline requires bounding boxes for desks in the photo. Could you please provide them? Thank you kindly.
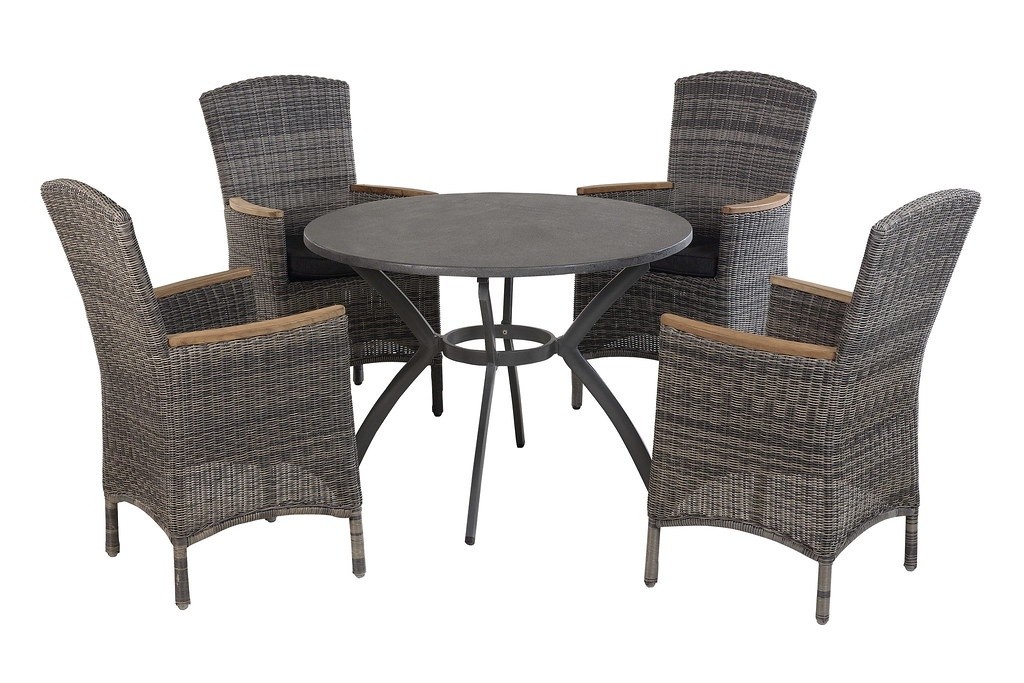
[303,192,692,545]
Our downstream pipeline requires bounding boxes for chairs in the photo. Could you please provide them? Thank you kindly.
[199,74,445,417]
[41,178,367,611]
[571,70,818,411]
[642,188,982,626]
